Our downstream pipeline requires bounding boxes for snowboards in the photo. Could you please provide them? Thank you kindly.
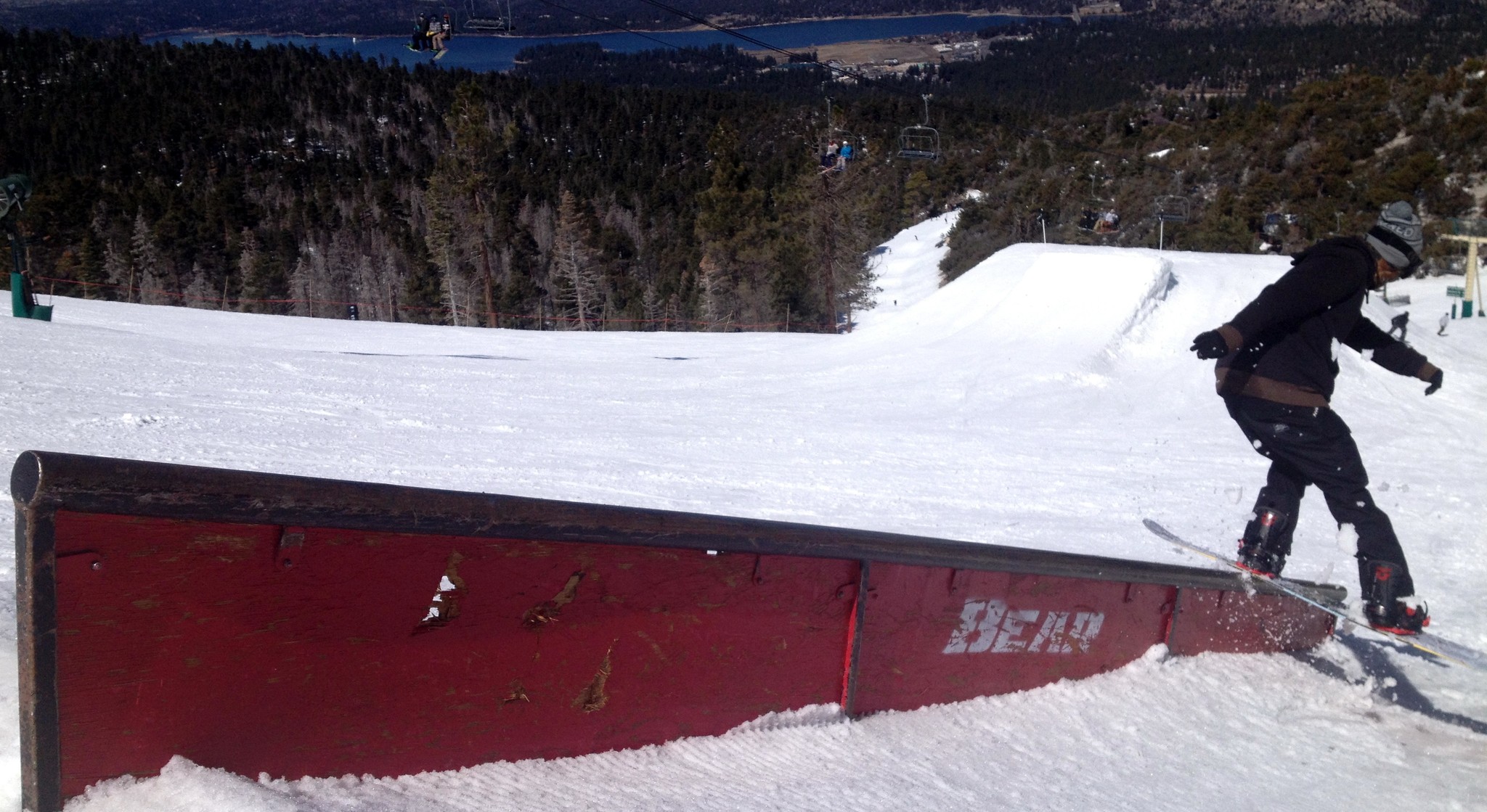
[1142,516,1487,680]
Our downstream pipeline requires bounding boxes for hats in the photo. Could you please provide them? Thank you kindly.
[1366,202,1424,269]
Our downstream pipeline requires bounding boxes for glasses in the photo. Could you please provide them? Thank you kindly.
[1388,265,1412,280]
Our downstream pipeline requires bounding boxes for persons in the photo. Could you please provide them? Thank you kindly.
[1437,313,1449,335]
[1079,206,1092,228]
[421,15,441,50]
[1100,209,1118,233]
[1087,207,1100,230]
[410,12,430,50]
[832,140,853,171]
[496,17,507,34]
[1093,208,1108,231]
[1386,311,1409,340]
[819,138,839,167]
[432,14,454,51]
[1189,199,1443,634]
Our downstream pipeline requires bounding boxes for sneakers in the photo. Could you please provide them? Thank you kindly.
[1236,541,1283,578]
[1366,599,1430,637]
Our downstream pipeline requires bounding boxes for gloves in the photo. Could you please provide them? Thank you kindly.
[1414,362,1443,395]
[1190,330,1228,360]
